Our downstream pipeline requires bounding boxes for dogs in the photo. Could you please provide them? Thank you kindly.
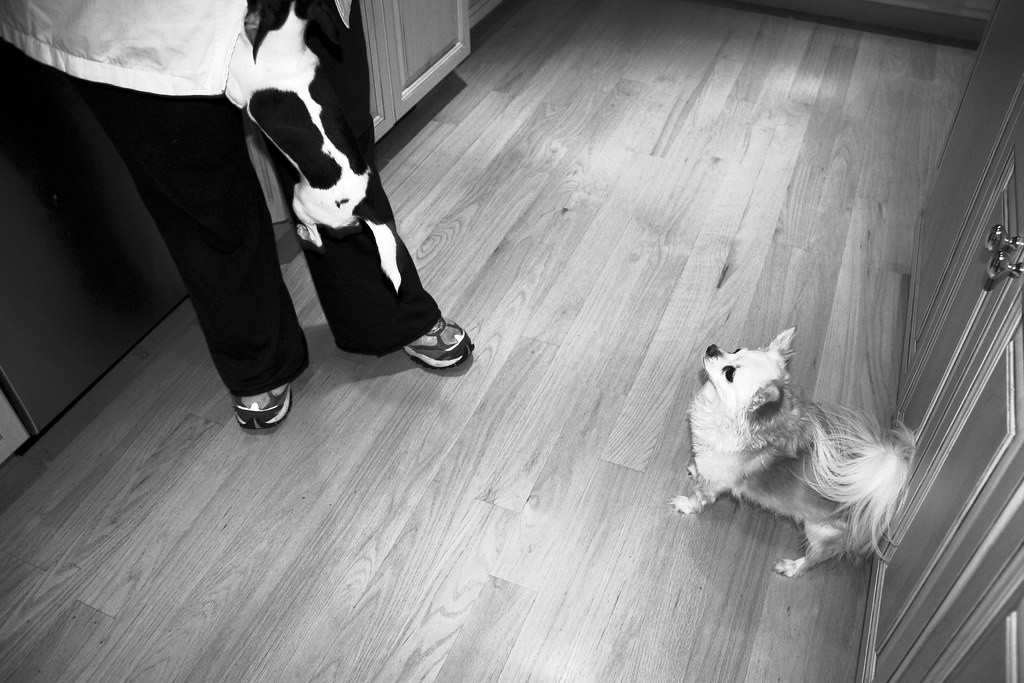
[671,326,915,582]
[224,0,403,296]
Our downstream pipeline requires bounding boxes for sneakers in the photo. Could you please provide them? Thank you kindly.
[229,382,294,430]
[402,315,475,370]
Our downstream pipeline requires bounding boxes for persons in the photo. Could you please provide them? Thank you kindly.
[0,0,473,431]
